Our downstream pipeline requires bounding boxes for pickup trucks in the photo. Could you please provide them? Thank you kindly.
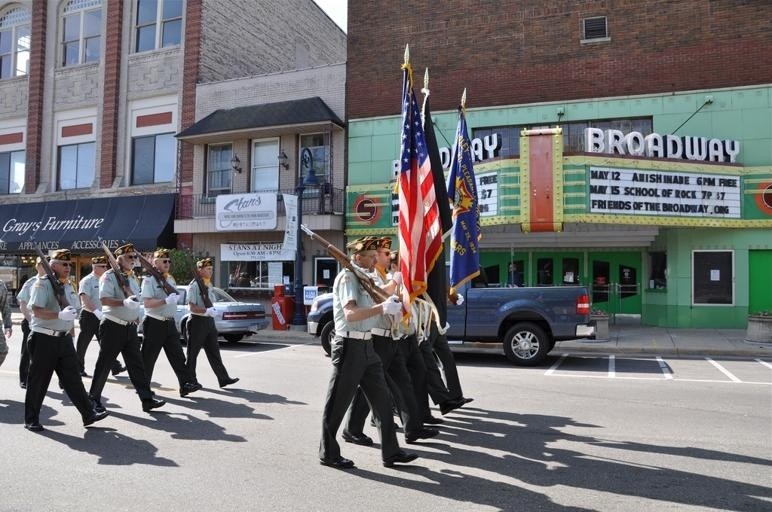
[306,260,598,367]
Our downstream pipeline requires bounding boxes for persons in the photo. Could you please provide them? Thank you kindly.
[23,247,112,432]
[317,236,476,471]
[17,255,54,388]
[87,243,168,413]
[186,256,239,387]
[0,279,13,367]
[140,249,203,396]
[76,254,127,377]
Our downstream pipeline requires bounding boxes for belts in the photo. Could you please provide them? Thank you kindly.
[105,314,137,328]
[32,326,69,338]
[336,327,408,341]
[145,312,172,322]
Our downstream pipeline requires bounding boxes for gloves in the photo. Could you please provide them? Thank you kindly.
[165,293,178,305]
[204,307,217,318]
[92,308,105,321]
[58,306,77,322]
[448,293,464,305]
[380,295,403,315]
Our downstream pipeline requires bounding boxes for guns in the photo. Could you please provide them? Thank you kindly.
[300,223,398,304]
[179,242,212,307]
[29,234,70,311]
[121,239,179,296]
[96,234,139,302]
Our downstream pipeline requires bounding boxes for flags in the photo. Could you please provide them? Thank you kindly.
[444,106,484,291]
[397,61,444,303]
[424,86,459,240]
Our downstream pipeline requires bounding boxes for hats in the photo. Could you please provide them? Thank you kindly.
[35,243,213,268]
[347,234,398,261]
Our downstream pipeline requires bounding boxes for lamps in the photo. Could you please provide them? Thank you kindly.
[231,154,241,172]
[278,152,288,169]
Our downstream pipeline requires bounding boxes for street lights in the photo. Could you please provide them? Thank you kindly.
[292,147,320,325]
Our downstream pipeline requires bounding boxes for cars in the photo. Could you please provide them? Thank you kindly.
[250,276,268,287]
[137,284,268,338]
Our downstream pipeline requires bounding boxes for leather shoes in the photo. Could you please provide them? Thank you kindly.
[112,366,126,375]
[83,406,111,426]
[24,421,44,431]
[180,381,202,397]
[142,398,166,412]
[220,377,239,387]
[320,396,473,469]
